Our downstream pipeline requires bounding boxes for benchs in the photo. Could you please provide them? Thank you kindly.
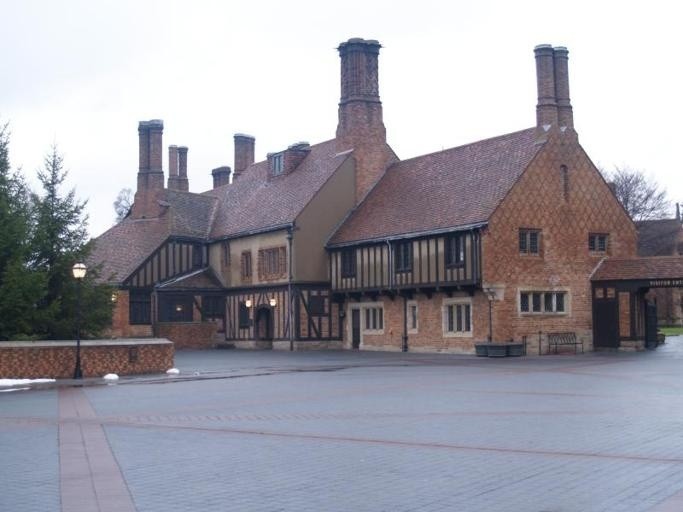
[547,330,583,355]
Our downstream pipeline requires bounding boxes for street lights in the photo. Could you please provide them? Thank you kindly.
[66,261,90,381]
